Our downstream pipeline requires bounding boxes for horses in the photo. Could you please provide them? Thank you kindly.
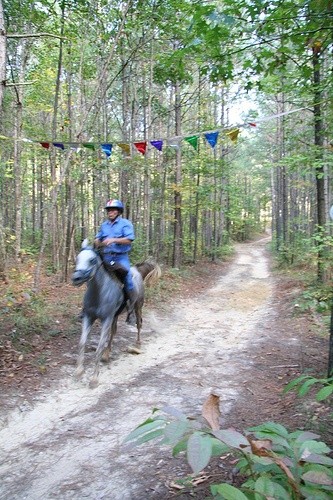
[71,239,161,389]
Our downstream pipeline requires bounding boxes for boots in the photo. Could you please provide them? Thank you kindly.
[126,300,136,324]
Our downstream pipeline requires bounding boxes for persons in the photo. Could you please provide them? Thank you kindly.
[79,199,137,325]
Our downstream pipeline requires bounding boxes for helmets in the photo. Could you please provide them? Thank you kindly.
[104,199,123,209]
